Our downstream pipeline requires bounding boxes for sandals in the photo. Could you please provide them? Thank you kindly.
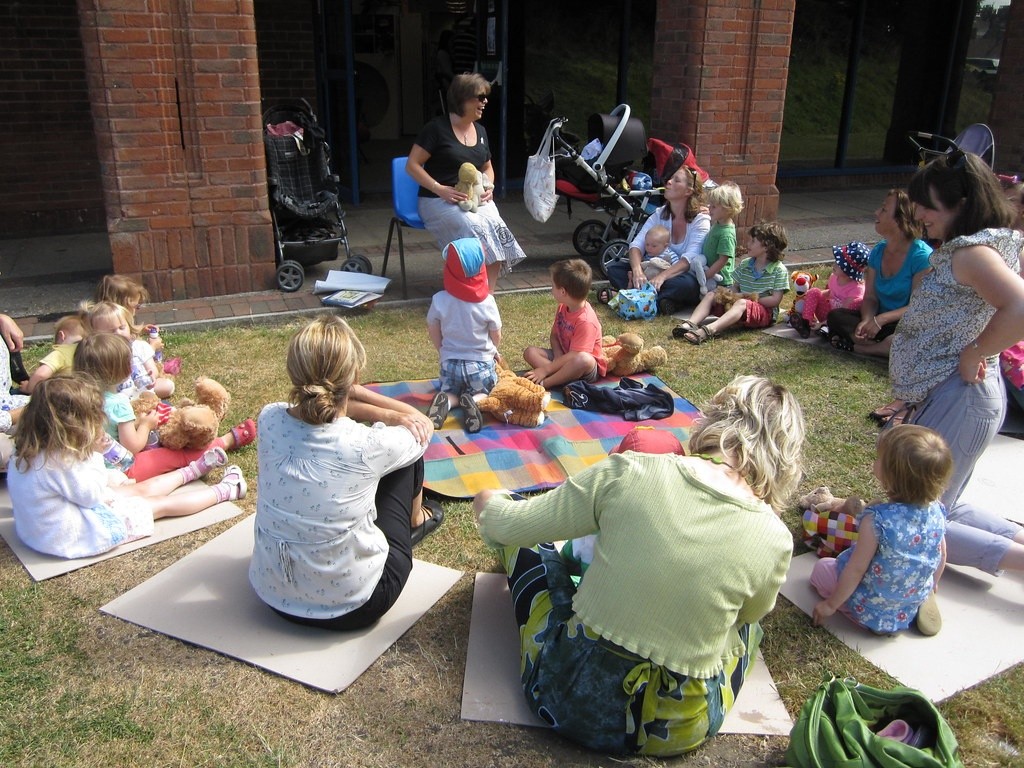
[672,321,698,336]
[223,463,248,502]
[189,447,228,480]
[597,288,619,304]
[683,325,714,344]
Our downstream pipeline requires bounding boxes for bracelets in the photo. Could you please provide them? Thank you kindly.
[971,339,986,359]
[431,181,437,192]
[874,316,882,330]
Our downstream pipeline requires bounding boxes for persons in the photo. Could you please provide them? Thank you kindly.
[249,314,444,632]
[827,189,934,358]
[889,153,1024,583]
[994,174,1023,417]
[557,425,685,589]
[810,424,955,637]
[789,241,871,343]
[626,224,680,290]
[425,238,503,434]
[0,273,256,560]
[473,372,807,758]
[702,180,745,288]
[523,259,607,388]
[405,72,527,296]
[672,219,791,345]
[597,164,712,315]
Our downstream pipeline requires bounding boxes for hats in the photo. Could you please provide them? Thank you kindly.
[608,425,686,464]
[444,242,490,302]
[832,241,872,281]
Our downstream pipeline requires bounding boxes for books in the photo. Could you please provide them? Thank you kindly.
[323,290,370,306]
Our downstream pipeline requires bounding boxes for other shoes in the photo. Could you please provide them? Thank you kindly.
[874,722,934,751]
[425,392,449,430]
[918,590,942,636]
[459,394,483,434]
[789,314,855,354]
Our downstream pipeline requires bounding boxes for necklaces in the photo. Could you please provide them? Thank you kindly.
[451,114,472,146]
[688,453,741,475]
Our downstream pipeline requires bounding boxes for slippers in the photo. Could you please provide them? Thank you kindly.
[229,419,257,451]
[880,410,913,429]
[868,403,898,418]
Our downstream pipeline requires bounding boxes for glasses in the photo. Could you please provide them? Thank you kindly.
[473,93,486,102]
[688,167,697,187]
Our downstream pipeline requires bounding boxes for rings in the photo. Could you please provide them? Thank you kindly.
[451,198,453,201]
[412,420,418,423]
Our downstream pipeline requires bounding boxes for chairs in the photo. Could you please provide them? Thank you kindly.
[380,157,427,300]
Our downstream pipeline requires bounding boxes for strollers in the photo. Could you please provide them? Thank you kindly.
[543,103,712,279]
[261,95,374,293]
[910,123,1000,191]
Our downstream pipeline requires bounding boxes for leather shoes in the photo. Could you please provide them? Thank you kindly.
[409,500,445,549]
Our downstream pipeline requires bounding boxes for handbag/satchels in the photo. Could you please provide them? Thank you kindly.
[522,119,563,225]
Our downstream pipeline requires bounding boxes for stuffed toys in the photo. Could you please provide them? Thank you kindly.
[474,363,552,427]
[713,286,759,315]
[600,333,668,377]
[640,257,672,280]
[799,486,869,517]
[690,254,723,300]
[472,170,490,206]
[783,271,820,327]
[454,162,477,213]
